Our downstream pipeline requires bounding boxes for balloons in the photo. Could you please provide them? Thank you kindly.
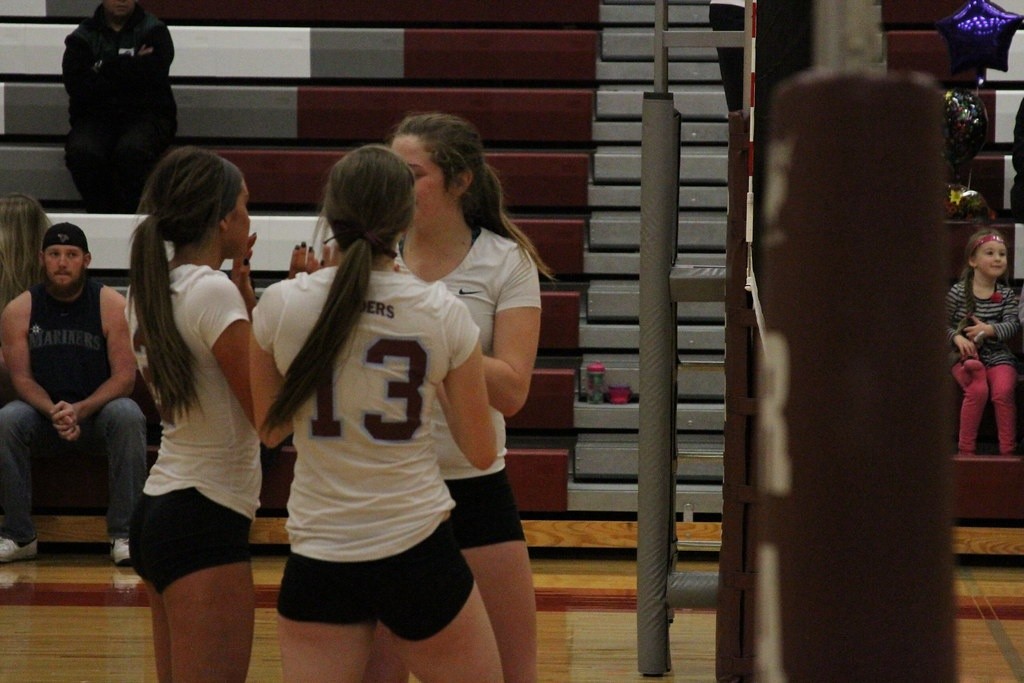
[936,0,1024,77]
[943,91,993,225]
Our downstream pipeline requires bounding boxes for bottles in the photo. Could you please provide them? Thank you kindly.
[586,360,605,404]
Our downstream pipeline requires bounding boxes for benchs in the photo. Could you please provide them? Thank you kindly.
[0,0,600,524]
[877,0,1024,518]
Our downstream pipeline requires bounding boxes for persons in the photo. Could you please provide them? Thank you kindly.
[129,114,554,683]
[0,193,146,565]
[941,227,1024,458]
[62,1,178,217]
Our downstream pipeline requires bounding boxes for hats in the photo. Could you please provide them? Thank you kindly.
[42,222,88,252]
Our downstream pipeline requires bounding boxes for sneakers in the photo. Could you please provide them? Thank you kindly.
[110,539,132,567]
[0,536,37,562]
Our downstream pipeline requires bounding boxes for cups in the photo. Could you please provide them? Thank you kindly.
[608,384,629,403]
